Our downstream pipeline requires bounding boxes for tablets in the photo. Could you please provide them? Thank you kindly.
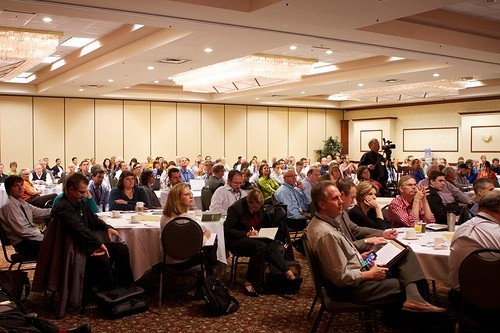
[372,239,409,268]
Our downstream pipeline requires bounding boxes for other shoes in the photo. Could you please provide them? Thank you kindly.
[287,275,303,286]
[240,283,259,297]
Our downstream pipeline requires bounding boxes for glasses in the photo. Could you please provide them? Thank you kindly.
[179,192,193,198]
[231,180,243,186]
[284,174,297,178]
[74,188,86,194]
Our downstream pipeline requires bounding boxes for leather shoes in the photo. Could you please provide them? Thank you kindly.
[402,300,446,313]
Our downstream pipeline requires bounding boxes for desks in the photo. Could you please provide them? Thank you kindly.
[375,197,397,217]
[385,225,464,296]
[0,182,46,208]
[95,209,226,305]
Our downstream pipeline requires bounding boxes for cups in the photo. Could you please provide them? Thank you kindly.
[406,230,419,239]
[131,215,139,222]
[112,211,120,217]
[415,221,422,234]
[422,223,426,233]
[137,202,143,215]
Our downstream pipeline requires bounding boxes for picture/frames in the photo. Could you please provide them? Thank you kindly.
[403,127,459,151]
[471,126,500,152]
[361,130,383,151]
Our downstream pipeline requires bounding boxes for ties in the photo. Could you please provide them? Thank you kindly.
[20,205,34,227]
[95,188,100,203]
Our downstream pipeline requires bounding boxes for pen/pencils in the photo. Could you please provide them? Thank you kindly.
[398,231,404,233]
[252,227,255,230]
[390,226,394,231]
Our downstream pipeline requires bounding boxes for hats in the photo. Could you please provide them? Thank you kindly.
[240,169,253,178]
[213,165,226,172]
[458,164,471,170]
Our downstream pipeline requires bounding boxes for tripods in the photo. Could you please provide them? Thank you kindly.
[376,158,400,197]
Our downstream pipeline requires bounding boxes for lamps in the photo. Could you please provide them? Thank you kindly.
[0,26,64,81]
[171,52,318,93]
[346,78,479,102]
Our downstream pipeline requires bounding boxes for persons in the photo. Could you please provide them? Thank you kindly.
[224,188,303,297]
[0,155,321,214]
[305,181,447,333]
[0,175,52,259]
[446,190,500,311]
[320,154,500,240]
[51,173,134,303]
[160,183,217,297]
[358,138,388,193]
[274,169,313,256]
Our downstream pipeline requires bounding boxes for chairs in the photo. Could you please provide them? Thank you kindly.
[449,249,500,333]
[0,204,295,313]
[302,237,378,333]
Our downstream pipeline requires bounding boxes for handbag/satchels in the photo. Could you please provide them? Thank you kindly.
[203,282,240,317]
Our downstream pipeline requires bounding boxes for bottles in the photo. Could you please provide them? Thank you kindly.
[448,211,454,232]
